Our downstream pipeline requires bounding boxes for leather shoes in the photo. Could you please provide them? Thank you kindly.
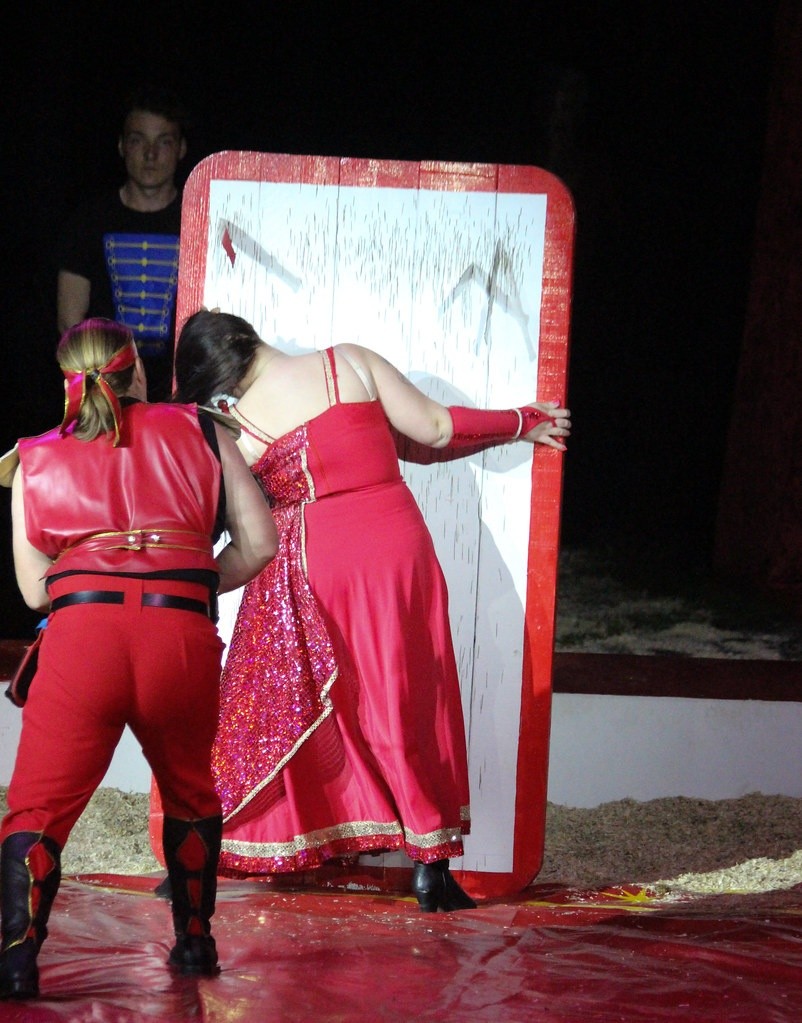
[170,937,218,973]
[0,948,39,998]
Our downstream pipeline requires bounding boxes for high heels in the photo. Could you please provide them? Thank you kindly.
[413,858,477,912]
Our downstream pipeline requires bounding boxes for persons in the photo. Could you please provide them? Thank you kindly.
[154,311,571,913]
[0,318,280,998]
[58,96,184,402]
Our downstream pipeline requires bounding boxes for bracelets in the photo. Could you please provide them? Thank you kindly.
[510,408,523,440]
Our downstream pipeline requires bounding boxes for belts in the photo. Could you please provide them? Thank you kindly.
[48,590,210,617]
